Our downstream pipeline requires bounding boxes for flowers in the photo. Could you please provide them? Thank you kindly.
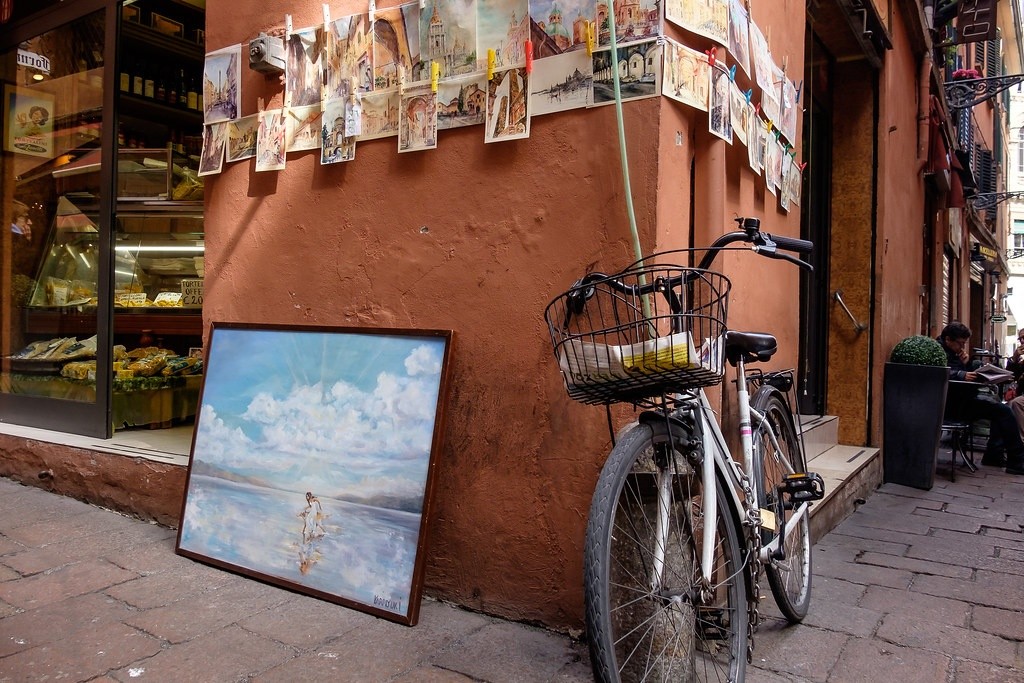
[952,68,981,83]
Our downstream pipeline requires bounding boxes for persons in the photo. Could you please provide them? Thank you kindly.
[1005,328,1024,443]
[936,321,1024,475]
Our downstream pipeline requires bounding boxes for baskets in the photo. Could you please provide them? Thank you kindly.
[546,267,733,407]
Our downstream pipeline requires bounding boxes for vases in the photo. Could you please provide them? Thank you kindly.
[955,78,975,93]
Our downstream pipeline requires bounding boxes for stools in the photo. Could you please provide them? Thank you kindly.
[941,422,975,482]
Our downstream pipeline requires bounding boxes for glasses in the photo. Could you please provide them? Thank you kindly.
[1017,336,1024,340]
[955,340,967,347]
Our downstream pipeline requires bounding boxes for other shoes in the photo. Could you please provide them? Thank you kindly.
[981,449,1006,467]
[1006,461,1024,475]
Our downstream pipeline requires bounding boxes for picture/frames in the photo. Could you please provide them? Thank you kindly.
[173,322,454,626]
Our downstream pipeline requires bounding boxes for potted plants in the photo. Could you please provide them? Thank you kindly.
[880,335,951,489]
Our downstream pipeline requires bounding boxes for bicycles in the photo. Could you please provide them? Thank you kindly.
[544,217,826,683]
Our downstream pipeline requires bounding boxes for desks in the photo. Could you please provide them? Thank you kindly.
[948,378,995,387]
[971,354,996,363]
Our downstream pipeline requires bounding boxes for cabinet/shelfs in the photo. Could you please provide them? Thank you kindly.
[25,17,205,339]
